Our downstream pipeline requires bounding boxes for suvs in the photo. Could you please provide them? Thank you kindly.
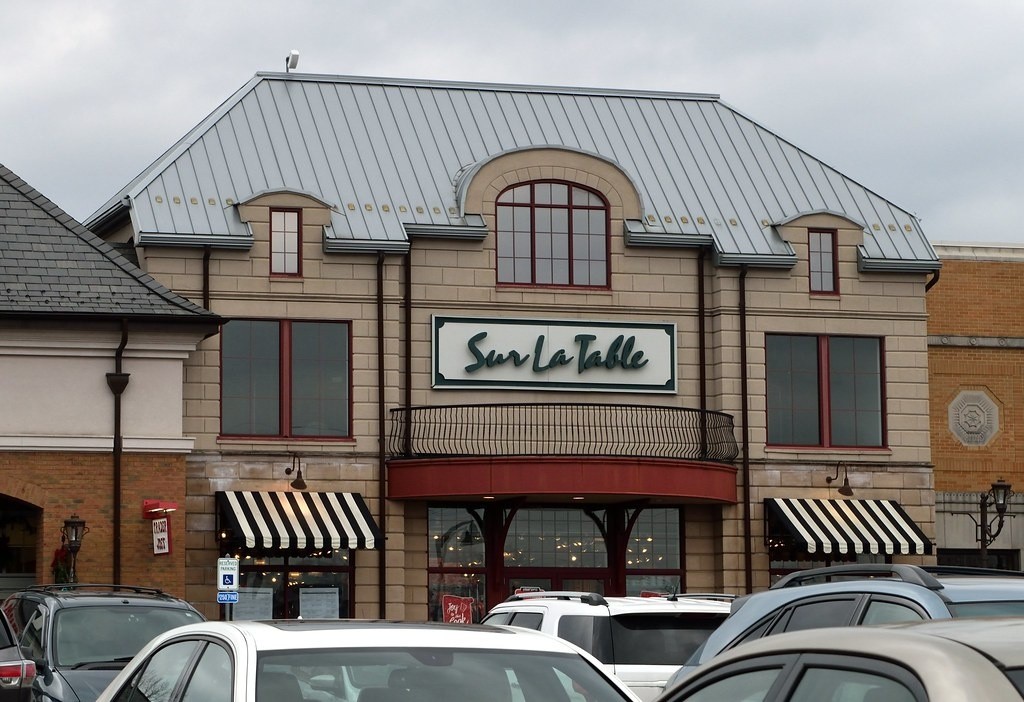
[661,563,1023,698]
[478,587,742,702]
[4,584,208,702]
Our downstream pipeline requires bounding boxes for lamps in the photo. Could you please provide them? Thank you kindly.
[285,451,306,490]
[826,461,854,496]
[284,49,299,72]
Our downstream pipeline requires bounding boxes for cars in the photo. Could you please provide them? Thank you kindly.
[0,607,37,702]
[652,615,1023,702]
[286,660,514,702]
[97,619,644,702]
